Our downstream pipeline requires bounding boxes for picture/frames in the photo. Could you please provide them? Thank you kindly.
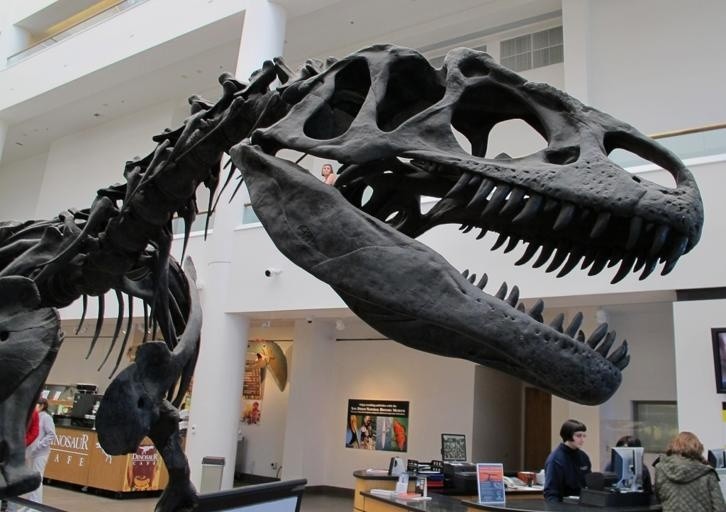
[709,326,725,395]
[631,399,678,454]
[475,462,506,505]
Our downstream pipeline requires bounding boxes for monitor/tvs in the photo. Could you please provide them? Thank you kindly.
[440,432,467,461]
[708,449,726,469]
[610,446,645,492]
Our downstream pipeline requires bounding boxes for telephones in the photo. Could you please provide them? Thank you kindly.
[503,476,516,488]
[388,456,404,476]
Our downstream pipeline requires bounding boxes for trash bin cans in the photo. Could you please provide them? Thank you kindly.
[200,456,225,494]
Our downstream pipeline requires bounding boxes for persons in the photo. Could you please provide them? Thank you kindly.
[443,438,466,459]
[543,419,593,504]
[320,163,337,186]
[27,397,56,503]
[346,413,407,449]
[25,408,39,447]
[652,430,726,512]
[606,435,652,489]
